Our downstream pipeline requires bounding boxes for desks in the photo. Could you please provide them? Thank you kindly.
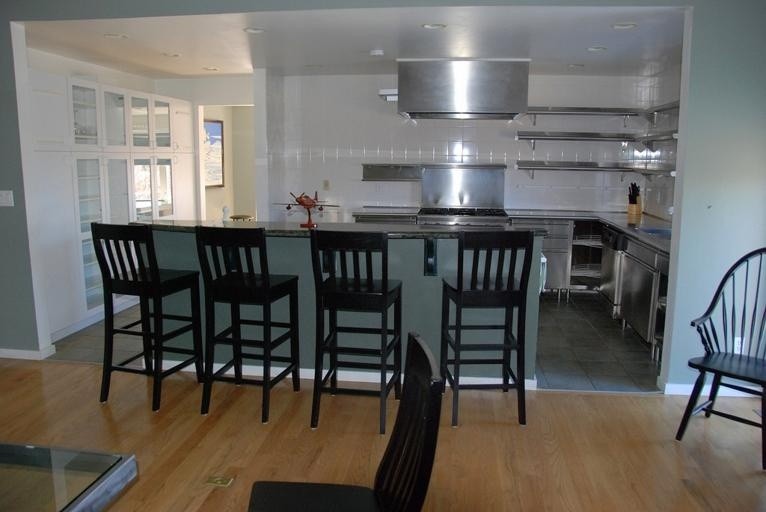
[0,442,140,512]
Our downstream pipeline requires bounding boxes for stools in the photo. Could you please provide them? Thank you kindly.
[229,214,254,222]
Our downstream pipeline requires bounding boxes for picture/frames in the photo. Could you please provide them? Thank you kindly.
[204,120,225,187]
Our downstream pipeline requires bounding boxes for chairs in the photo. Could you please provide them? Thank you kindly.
[196,226,301,424]
[309,229,401,435]
[90,222,204,413]
[248,331,444,512]
[676,247,765,470]
[440,230,535,430]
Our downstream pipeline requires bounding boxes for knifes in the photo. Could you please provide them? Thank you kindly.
[628,182,640,203]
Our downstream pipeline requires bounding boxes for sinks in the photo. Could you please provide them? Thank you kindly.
[638,226,672,234]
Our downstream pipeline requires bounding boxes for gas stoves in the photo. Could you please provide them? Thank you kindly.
[417,208,510,225]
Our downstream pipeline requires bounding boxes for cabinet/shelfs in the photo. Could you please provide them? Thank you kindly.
[517,99,678,176]
[513,219,670,364]
[29,72,198,334]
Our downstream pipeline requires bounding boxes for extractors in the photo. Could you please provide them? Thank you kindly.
[396,60,529,119]
